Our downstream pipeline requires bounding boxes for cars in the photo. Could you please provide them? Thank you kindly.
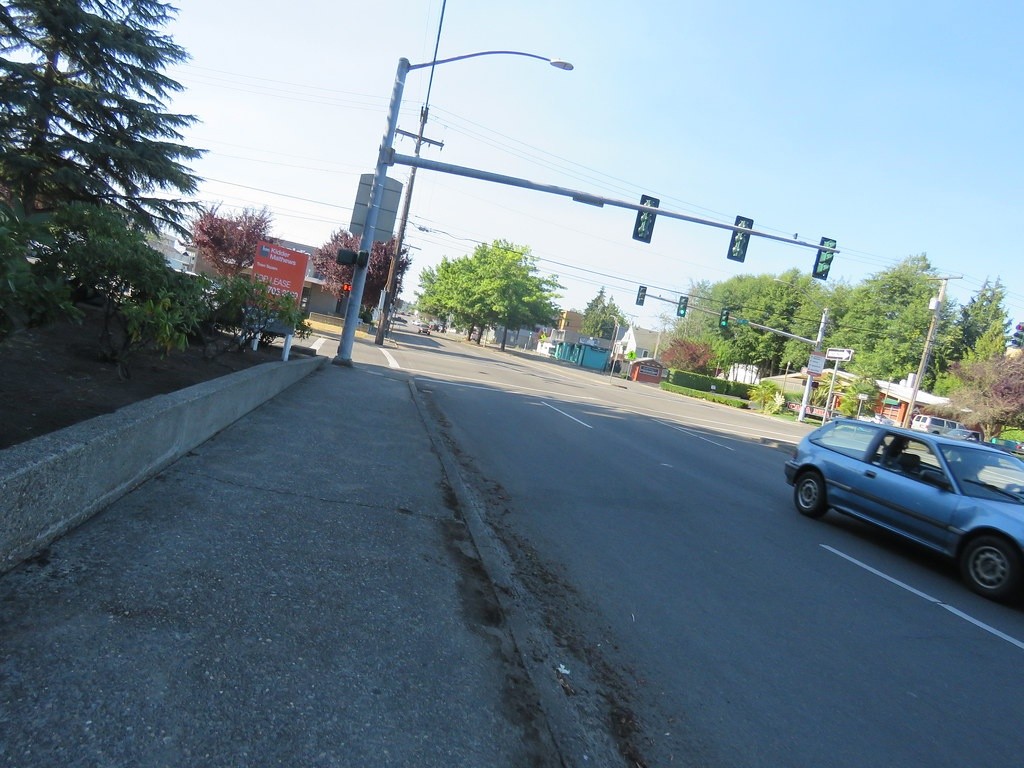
[392,316,408,324]
[418,323,431,335]
[427,321,450,334]
[410,319,420,326]
[783,417,1024,605]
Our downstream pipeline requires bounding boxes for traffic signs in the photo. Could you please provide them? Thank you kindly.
[825,348,854,362]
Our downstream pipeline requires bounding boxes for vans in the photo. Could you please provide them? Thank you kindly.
[911,414,968,436]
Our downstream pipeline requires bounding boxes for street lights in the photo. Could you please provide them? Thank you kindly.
[774,277,831,421]
[333,49,575,370]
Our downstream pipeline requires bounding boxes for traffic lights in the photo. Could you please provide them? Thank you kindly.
[676,296,689,318]
[1011,321,1024,349]
[718,307,730,329]
[635,285,648,306]
[342,282,352,292]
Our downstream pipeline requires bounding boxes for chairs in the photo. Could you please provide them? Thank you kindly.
[920,469,945,484]
[901,452,920,476]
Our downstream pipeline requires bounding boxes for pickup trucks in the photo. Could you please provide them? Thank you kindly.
[944,428,1012,455]
